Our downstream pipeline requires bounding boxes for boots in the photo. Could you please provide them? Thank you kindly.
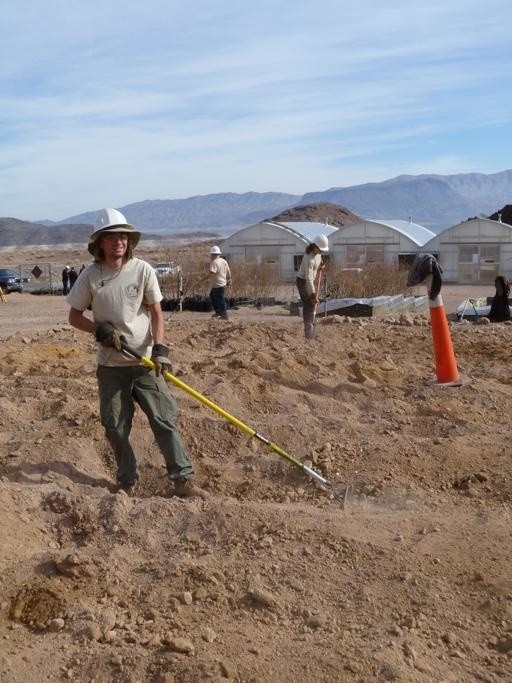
[116,479,141,498]
[174,478,213,501]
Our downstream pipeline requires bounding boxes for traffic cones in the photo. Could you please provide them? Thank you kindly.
[424,261,474,387]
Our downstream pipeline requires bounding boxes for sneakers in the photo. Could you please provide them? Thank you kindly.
[212,312,229,320]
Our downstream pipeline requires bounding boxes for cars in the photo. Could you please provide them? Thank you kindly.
[155,260,183,276]
[0,268,31,292]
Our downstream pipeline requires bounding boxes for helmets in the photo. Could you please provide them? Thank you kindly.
[90,207,133,239]
[208,246,221,255]
[315,234,329,251]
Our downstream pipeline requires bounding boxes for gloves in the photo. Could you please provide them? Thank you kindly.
[150,343,175,380]
[310,293,319,304]
[95,320,128,353]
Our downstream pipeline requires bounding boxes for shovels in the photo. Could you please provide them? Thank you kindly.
[304,263,325,339]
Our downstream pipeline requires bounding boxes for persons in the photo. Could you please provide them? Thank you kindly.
[296,233,330,340]
[78,263,86,274]
[485,275,510,322]
[61,265,69,295]
[201,245,232,320]
[64,207,211,500]
[67,266,77,290]
